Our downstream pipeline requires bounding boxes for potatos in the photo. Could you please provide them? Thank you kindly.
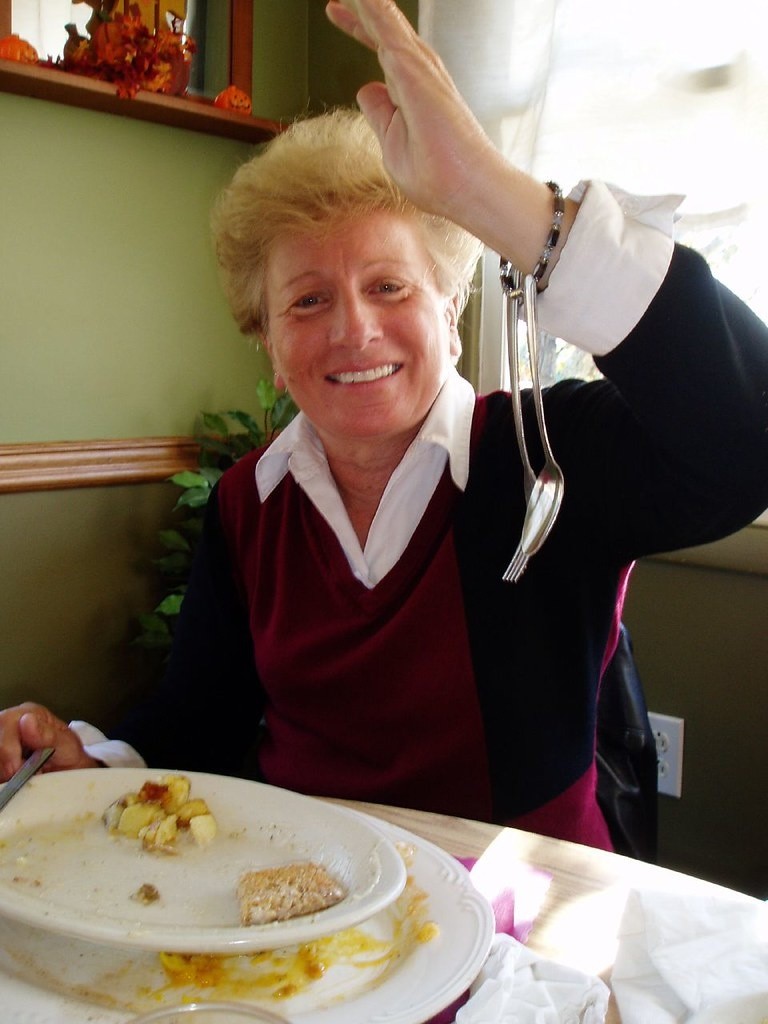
[102,774,216,850]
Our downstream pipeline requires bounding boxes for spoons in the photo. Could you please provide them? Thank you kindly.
[520,275,565,559]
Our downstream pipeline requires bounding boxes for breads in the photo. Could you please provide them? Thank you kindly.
[240,863,345,925]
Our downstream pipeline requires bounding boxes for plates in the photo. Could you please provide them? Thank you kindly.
[0,801,496,1024]
[1,768,408,956]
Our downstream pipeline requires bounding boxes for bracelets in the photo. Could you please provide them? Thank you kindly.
[498,181,565,300]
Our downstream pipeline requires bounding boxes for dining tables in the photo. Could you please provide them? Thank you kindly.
[307,796,768,1024]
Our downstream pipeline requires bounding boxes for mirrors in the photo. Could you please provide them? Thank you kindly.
[0,0,254,115]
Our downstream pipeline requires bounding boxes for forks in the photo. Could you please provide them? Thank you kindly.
[499,266,540,582]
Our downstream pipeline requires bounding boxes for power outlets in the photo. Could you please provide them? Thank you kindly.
[647,712,684,799]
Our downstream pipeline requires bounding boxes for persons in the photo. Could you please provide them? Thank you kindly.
[0,0,768,853]
